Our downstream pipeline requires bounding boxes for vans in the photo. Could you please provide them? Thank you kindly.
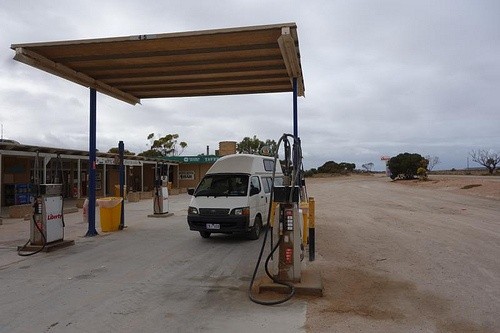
[187,154,283,241]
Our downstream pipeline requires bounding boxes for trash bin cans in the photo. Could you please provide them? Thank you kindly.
[299,202,309,247]
[115,184,127,200]
[168,182,173,195]
[97,196,124,232]
